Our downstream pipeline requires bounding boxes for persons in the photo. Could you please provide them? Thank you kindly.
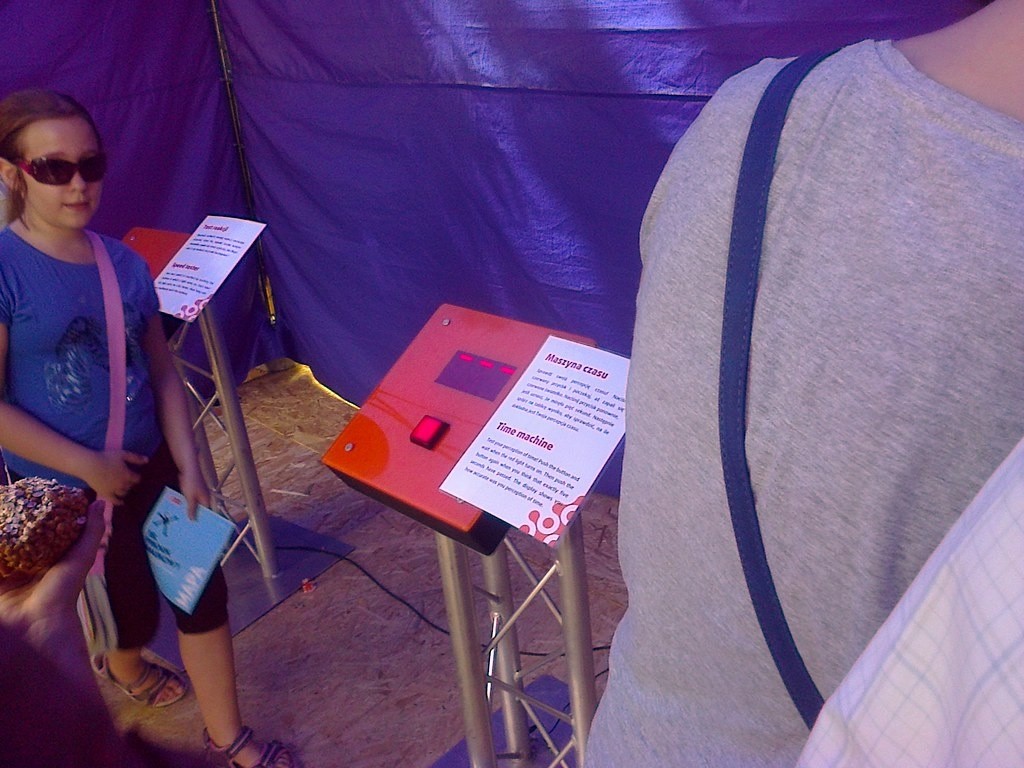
[797,437,1024,768]
[0,87,300,768]
[584,0,1024,768]
[0,485,212,768]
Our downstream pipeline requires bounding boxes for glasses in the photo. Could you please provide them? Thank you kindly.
[17,155,107,185]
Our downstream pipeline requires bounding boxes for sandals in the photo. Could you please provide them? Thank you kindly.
[89,650,187,707]
[203,725,297,768]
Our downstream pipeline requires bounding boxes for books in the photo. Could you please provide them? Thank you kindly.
[142,488,237,614]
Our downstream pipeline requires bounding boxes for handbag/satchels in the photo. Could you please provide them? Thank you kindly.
[76,547,118,657]
